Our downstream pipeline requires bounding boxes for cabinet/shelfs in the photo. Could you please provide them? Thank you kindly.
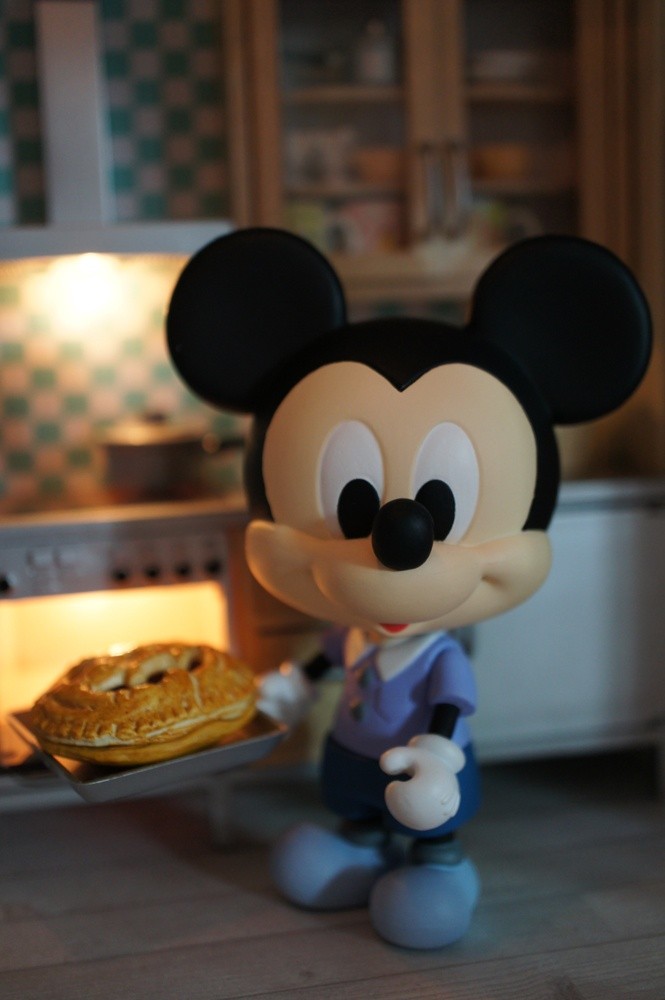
[0,0,244,253]
[224,0,644,308]
[444,484,665,767]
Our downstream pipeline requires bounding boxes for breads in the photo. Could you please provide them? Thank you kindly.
[26,642,257,767]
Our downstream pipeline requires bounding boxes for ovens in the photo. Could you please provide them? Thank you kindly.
[2,490,328,811]
